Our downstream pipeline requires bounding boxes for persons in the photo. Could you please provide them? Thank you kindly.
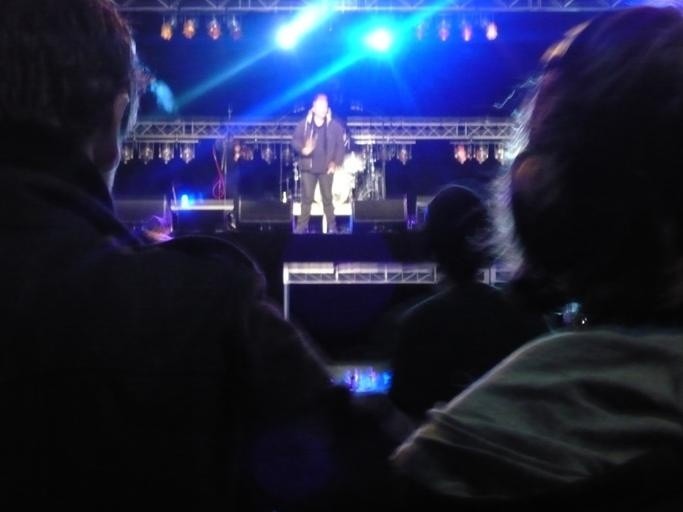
[0,0,387,511]
[290,94,345,235]
[378,185,549,433]
[383,6,683,512]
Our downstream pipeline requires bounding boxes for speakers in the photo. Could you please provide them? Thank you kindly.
[235,200,293,233]
[415,196,434,222]
[352,199,408,235]
[171,201,234,237]
